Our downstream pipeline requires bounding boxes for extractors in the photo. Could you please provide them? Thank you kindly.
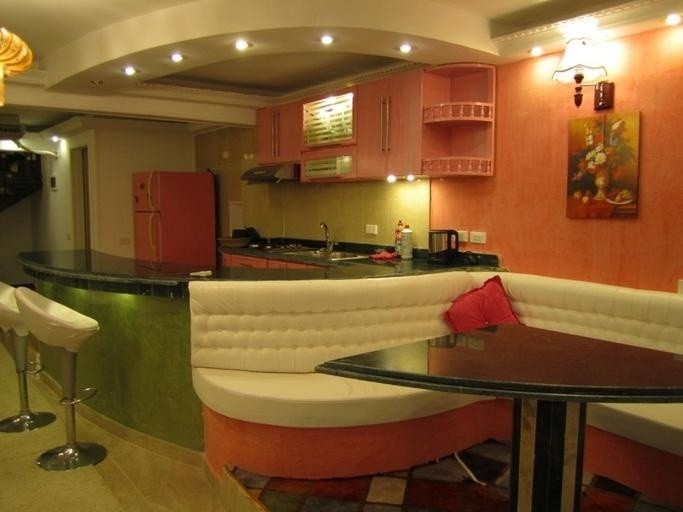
[239,163,298,185]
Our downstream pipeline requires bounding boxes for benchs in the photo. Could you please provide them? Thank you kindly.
[190,266,681,511]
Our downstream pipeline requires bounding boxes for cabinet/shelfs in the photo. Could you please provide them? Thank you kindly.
[421,62,498,183]
[352,60,421,180]
[250,97,303,181]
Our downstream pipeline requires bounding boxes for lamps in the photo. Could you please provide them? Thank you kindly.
[0,24,36,106]
[549,38,610,111]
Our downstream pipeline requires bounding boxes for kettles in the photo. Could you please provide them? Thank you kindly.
[425,229,459,264]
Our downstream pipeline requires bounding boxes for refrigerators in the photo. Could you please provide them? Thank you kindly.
[130,169,217,268]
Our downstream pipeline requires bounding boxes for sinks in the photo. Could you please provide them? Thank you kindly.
[284,249,369,263]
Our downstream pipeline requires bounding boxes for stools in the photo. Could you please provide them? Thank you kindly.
[15,282,111,469]
[0,283,53,432]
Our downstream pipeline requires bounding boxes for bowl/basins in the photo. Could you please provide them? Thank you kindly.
[217,236,251,248]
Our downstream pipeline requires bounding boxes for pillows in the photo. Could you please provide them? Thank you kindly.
[442,276,523,332]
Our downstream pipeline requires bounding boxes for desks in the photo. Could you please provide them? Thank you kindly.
[311,318,680,512]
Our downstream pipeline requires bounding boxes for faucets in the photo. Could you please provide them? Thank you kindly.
[319,221,331,246]
